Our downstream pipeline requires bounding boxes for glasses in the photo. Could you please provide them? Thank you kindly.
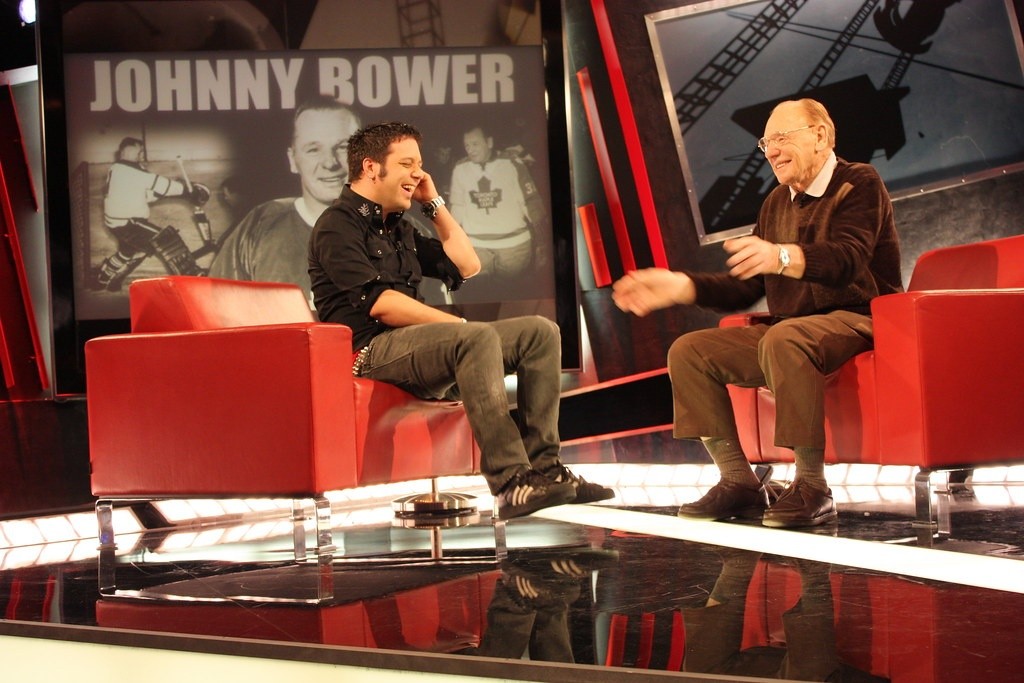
[757,124,815,151]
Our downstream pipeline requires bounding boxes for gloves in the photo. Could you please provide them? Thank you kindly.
[182,180,209,202]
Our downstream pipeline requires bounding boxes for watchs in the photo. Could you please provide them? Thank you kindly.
[420,197,445,217]
[777,244,789,275]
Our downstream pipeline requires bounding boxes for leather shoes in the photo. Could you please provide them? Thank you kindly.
[677,480,770,523]
[761,476,837,525]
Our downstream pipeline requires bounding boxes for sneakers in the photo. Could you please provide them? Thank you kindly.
[527,547,620,585]
[497,469,575,520]
[498,566,579,600]
[539,460,616,504]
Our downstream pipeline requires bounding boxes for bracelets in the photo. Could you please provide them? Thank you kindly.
[462,318,467,323]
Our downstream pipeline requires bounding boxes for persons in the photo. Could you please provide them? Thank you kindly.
[93,136,209,291]
[309,123,615,521]
[209,101,360,311]
[612,98,906,529]
[448,124,547,278]
[680,541,841,683]
[478,548,619,663]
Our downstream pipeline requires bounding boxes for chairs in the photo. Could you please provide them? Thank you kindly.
[737,539,1024,683]
[720,235,1024,538]
[86,274,478,554]
[95,553,508,652]
